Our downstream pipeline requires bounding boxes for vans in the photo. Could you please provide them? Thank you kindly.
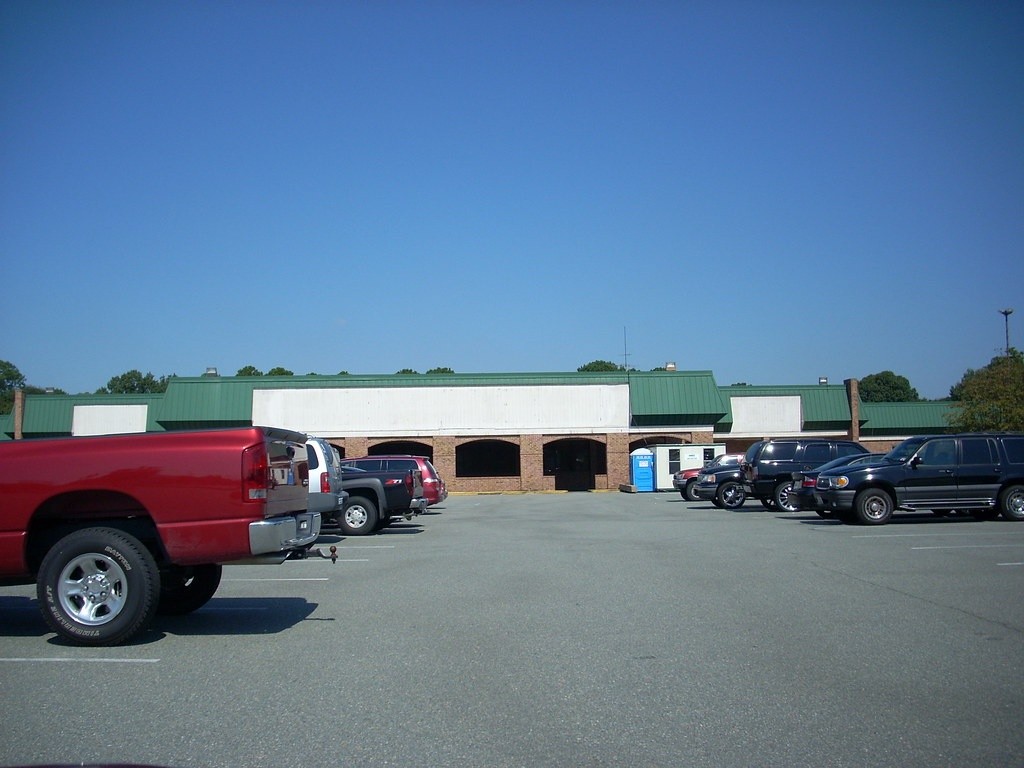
[340,455,448,506]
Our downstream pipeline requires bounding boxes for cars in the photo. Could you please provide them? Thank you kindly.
[787,452,889,519]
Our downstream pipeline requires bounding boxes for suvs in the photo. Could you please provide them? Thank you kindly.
[672,452,746,501]
[813,431,1024,525]
[693,464,754,509]
[735,438,871,512]
[304,435,350,512]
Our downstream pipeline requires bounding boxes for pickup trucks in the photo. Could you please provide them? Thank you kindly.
[1,426,310,647]
[336,466,416,535]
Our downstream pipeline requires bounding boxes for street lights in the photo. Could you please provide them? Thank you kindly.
[1000,308,1013,358]
